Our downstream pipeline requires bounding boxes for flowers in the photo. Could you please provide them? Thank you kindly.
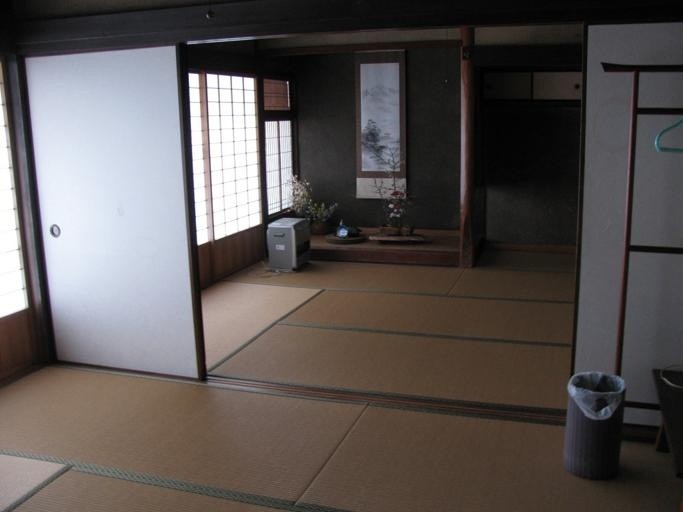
[364,117,419,230]
[283,171,341,221]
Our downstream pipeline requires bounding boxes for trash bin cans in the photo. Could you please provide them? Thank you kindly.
[266,218,311,272]
[563,372,628,479]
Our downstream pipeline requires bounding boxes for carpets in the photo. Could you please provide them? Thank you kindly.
[0,445,73,512]
[202,280,328,372]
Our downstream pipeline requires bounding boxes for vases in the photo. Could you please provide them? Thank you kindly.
[309,222,331,236]
[377,223,414,237]
